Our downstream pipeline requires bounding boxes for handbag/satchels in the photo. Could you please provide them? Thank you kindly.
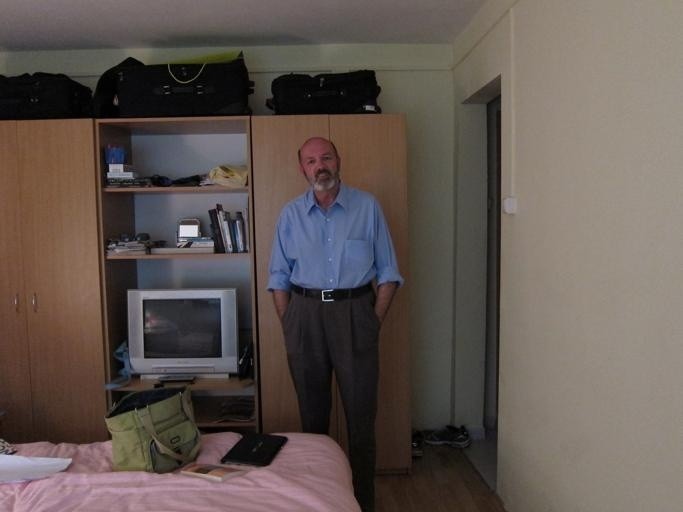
[103,384,203,473]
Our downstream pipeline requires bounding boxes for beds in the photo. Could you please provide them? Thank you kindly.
[0,432,367,512]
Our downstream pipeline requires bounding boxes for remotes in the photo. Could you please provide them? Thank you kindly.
[159,375,196,382]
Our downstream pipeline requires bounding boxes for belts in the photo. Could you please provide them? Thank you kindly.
[289,283,371,303]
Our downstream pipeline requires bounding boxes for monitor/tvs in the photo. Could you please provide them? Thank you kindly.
[126,287,240,380]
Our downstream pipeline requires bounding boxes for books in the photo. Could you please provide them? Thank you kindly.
[180,462,244,483]
[104,203,249,256]
[108,177,153,188]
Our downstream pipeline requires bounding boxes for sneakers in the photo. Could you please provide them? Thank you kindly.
[411,429,426,458]
[424,424,474,450]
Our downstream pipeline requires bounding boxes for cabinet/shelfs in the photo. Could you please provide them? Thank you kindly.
[0,116,109,446]
[90,112,256,438]
[249,110,415,484]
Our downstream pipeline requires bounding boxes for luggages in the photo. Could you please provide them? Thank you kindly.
[265,68,383,115]
[0,72,94,120]
[94,55,257,118]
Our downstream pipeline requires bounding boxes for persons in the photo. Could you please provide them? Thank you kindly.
[265,138,403,503]
